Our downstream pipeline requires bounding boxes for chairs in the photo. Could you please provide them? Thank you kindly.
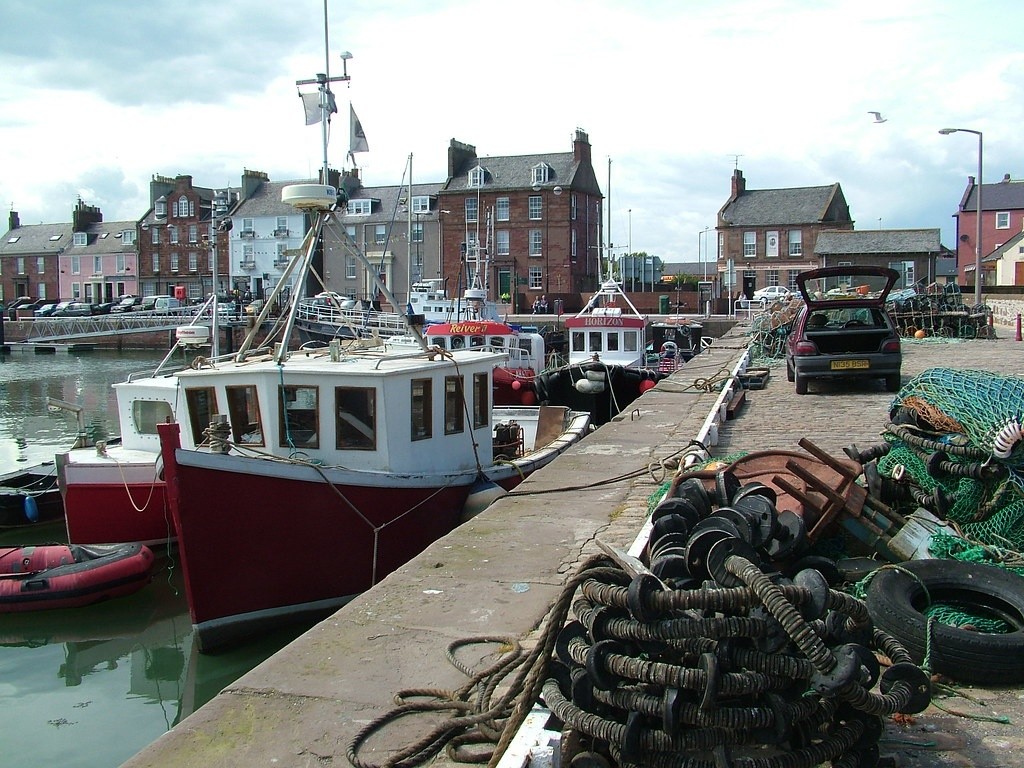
[810,314,829,327]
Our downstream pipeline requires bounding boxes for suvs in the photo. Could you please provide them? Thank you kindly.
[131,294,170,311]
[110,296,144,314]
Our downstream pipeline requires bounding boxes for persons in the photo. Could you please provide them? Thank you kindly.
[739,291,748,320]
[784,288,823,301]
[532,292,599,315]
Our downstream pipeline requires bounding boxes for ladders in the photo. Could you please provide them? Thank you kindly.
[658,342,678,374]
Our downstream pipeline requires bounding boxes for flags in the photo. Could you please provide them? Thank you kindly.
[303,92,336,125]
[350,102,369,152]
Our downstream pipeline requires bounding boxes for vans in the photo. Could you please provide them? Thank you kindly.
[51,303,102,316]
[155,298,186,316]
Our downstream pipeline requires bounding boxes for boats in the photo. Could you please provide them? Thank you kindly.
[0,2,716,657]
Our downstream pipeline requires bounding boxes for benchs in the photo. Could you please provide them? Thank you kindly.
[531,304,549,314]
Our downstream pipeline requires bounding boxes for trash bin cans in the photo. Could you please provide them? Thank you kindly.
[554,299,563,316]
[659,294,669,314]
[8,308,17,321]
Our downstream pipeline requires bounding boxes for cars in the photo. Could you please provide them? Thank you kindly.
[315,291,353,306]
[95,301,118,314]
[33,304,58,318]
[56,300,79,311]
[753,285,799,305]
[775,265,902,393]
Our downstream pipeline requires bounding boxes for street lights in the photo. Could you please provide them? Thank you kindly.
[628,209,633,256]
[704,226,709,318]
[936,127,984,309]
